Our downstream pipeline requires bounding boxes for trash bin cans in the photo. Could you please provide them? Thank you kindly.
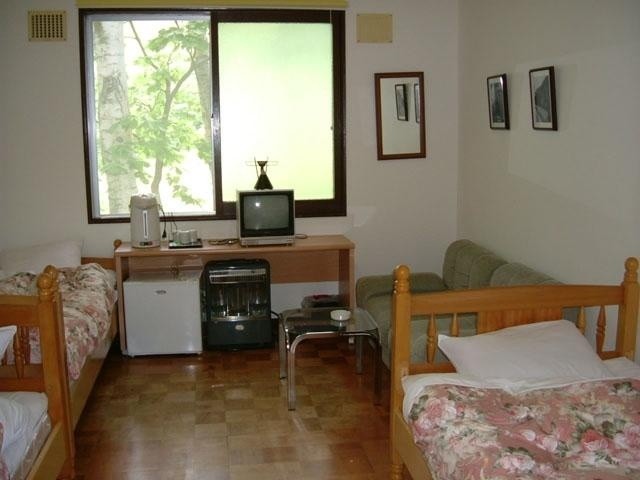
[304,294,346,332]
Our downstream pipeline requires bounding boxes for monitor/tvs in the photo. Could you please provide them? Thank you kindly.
[236,189,295,246]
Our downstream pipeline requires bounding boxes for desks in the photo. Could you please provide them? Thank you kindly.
[114,231,357,359]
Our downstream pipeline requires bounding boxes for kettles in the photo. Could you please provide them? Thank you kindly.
[129,195,164,249]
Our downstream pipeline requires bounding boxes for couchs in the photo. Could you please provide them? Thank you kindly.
[357,237,581,374]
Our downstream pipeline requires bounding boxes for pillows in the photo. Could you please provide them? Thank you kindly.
[1,237,85,276]
[1,323,19,361]
[436,316,616,388]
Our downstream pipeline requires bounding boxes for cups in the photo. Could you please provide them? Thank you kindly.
[171,229,199,246]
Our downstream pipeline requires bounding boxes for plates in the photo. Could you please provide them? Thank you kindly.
[168,238,204,248]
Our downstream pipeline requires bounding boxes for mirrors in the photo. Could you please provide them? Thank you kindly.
[374,70,427,162]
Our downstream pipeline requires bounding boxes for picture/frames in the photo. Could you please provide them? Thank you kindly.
[414,82,420,124]
[486,72,511,132]
[393,82,409,122]
[527,64,559,132]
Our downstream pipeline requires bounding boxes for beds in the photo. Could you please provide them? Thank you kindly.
[1,270,76,479]
[387,254,640,478]
[1,256,117,431]
